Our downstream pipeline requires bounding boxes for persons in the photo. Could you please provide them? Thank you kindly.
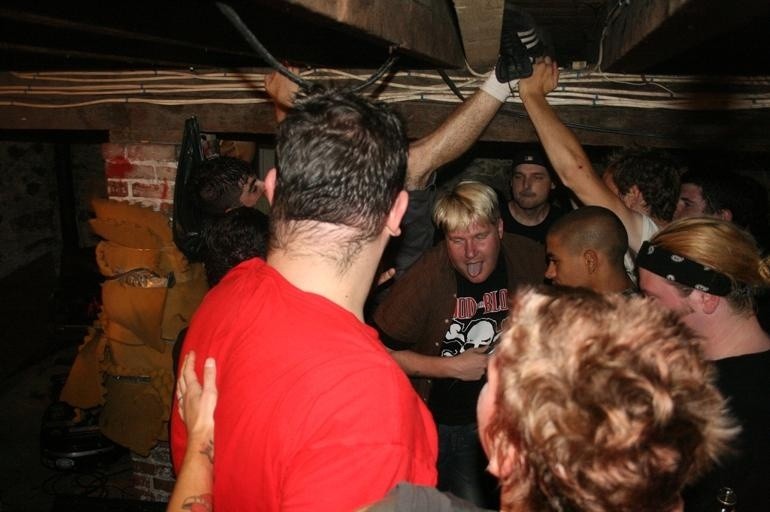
[163,5,755,512]
[634,217,769,510]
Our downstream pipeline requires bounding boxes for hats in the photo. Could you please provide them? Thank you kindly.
[511,148,554,178]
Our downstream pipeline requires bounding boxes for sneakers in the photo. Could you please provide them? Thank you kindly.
[495,2,548,83]
